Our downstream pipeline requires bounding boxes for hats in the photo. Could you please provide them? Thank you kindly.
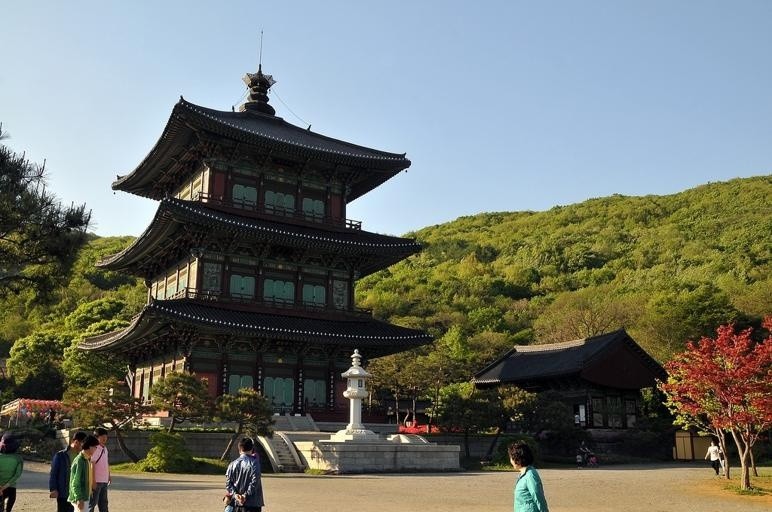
[0,434,19,452]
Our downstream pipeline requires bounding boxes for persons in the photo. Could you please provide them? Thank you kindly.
[507,440,549,512]
[705,442,721,474]
[0,431,23,512]
[49,431,87,512]
[67,436,100,512]
[719,443,726,471]
[577,440,591,456]
[575,411,581,427]
[224,437,265,512]
[87,428,112,512]
[46,408,55,423]
[577,453,584,470]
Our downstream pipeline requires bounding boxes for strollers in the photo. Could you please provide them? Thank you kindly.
[585,452,600,469]
